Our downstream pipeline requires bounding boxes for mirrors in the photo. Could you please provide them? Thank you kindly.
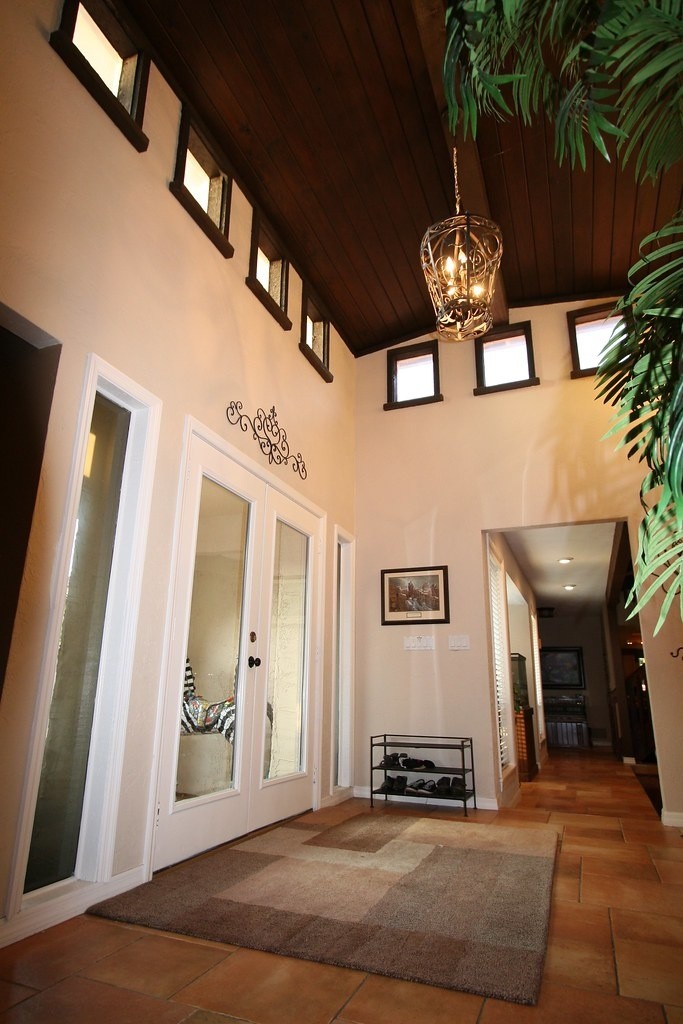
[22,388,343,895]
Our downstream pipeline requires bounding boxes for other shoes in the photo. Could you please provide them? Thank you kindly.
[377,752,435,770]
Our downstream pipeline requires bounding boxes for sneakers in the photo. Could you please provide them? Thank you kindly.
[380,775,408,795]
[405,778,437,795]
[436,776,467,797]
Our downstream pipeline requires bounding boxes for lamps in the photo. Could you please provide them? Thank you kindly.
[420,145,503,344]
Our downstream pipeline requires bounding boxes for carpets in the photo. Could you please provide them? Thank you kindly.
[85,810,558,1006]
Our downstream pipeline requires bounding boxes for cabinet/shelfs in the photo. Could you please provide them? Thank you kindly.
[369,734,478,817]
[515,706,540,782]
[543,700,592,748]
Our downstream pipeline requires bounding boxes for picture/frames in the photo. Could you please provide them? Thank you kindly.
[538,646,586,691]
[380,565,451,627]
